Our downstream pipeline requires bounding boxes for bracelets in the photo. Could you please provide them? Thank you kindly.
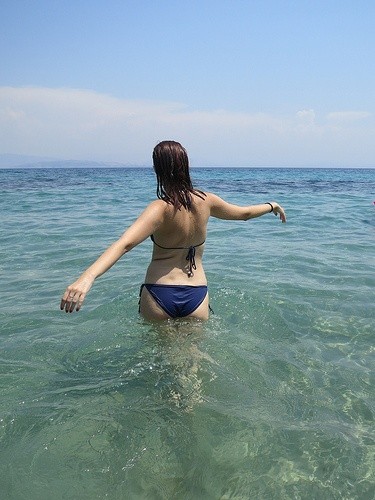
[265,202,273,213]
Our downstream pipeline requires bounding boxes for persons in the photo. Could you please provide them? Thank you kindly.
[60,140,286,320]
